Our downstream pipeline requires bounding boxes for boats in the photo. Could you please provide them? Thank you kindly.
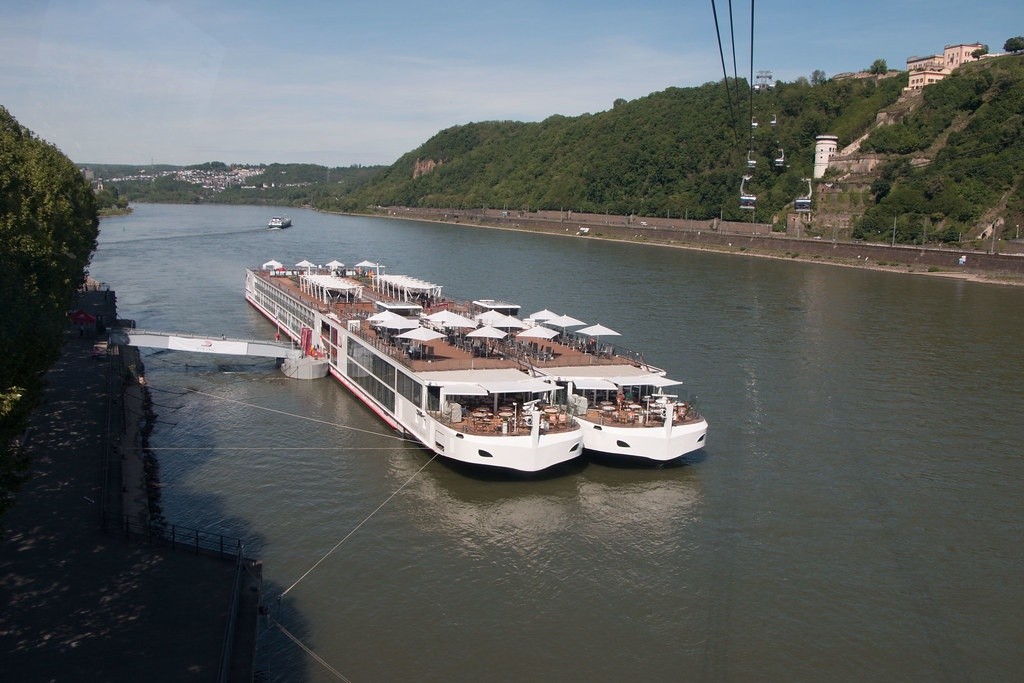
[269,214,291,228]
[243,261,707,477]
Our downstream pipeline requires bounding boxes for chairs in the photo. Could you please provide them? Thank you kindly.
[446,329,494,358]
[546,403,568,429]
[558,331,614,359]
[499,338,554,363]
[598,400,689,424]
[374,326,435,361]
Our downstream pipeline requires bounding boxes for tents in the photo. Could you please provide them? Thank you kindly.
[299,274,442,304]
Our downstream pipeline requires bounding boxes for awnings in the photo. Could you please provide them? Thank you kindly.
[443,377,683,413]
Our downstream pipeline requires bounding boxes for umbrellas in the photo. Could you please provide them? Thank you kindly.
[366,308,622,360]
[295,259,375,274]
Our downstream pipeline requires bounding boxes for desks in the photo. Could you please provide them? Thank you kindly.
[473,398,559,421]
[601,398,684,418]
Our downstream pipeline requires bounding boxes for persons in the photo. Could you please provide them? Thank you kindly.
[421,293,431,311]
[589,336,595,345]
[616,390,626,411]
[337,267,347,277]
[221,333,226,341]
